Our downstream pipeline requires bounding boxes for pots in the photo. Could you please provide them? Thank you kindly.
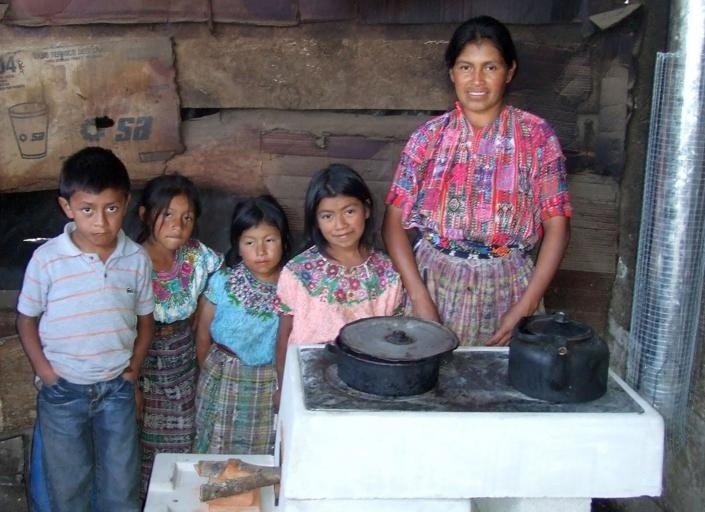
[326,315,458,397]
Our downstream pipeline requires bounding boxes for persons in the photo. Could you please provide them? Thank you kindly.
[12,146,154,511]
[275,163,405,414]
[382,18,570,345]
[131,172,224,508]
[191,195,291,454]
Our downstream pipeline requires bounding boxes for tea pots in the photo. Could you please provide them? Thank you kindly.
[506,311,607,403]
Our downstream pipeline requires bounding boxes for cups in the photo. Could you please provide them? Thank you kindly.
[8,102,49,160]
